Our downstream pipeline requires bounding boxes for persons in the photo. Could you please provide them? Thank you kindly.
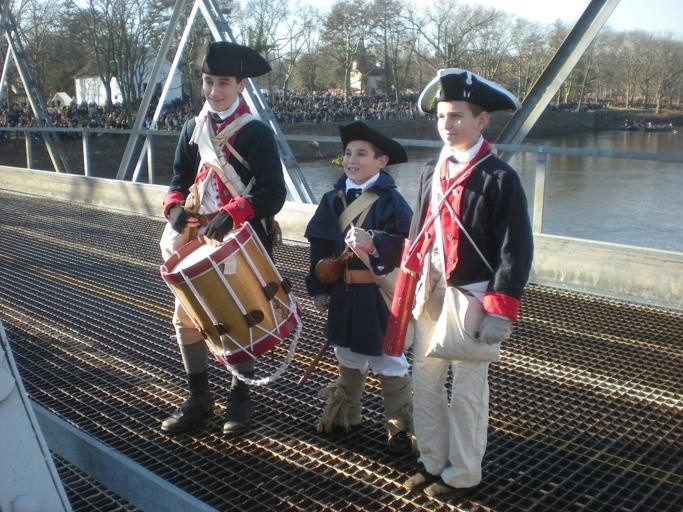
[160,41,287,436]
[400,69,534,497]
[302,121,415,454]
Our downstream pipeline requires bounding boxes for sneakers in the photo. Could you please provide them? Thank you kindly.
[158,391,213,433]
[388,430,412,454]
[401,469,438,493]
[422,478,475,497]
[322,422,360,434]
[220,391,253,434]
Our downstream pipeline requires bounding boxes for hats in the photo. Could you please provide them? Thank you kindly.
[337,121,409,167]
[417,66,522,118]
[199,40,272,78]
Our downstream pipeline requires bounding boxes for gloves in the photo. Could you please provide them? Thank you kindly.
[477,316,512,346]
[165,201,191,235]
[202,209,234,243]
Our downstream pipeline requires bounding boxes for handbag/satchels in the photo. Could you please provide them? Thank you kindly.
[424,285,501,363]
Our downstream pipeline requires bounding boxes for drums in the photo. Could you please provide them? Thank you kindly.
[159,219,303,366]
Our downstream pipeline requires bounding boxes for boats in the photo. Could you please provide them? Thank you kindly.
[624,121,640,131]
[643,122,671,132]
